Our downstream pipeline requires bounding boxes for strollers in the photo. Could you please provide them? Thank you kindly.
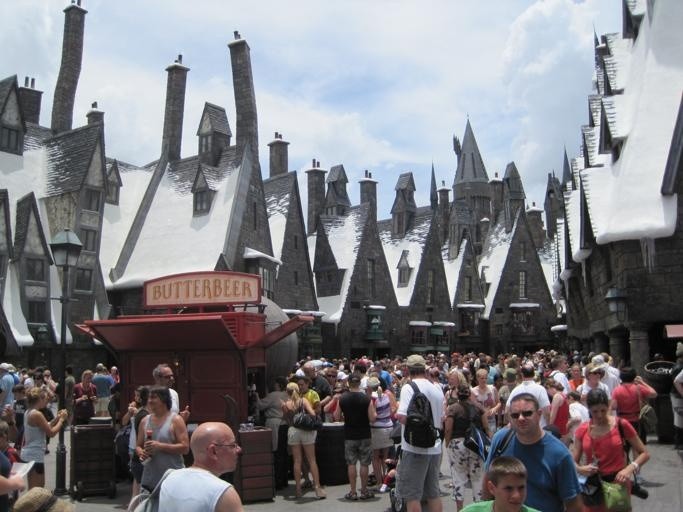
[384,424,405,510]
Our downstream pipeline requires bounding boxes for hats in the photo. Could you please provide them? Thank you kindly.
[0,363,9,371]
[337,372,349,379]
[13,487,75,512]
[585,363,602,379]
[276,376,288,385]
[501,368,518,381]
[592,355,609,368]
[407,354,426,368]
[8,364,15,372]
[368,377,381,387]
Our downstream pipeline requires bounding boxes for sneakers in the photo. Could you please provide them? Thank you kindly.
[636,475,643,484]
[380,484,387,493]
[345,491,358,501]
[296,492,302,499]
[316,489,327,499]
[359,491,375,500]
[301,481,312,491]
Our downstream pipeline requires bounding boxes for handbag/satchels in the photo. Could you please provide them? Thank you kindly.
[293,397,324,431]
[464,402,491,462]
[113,414,132,464]
[127,469,173,512]
[587,420,632,511]
[636,383,658,432]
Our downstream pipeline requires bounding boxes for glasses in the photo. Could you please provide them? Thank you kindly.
[326,373,337,378]
[509,409,537,419]
[33,377,42,380]
[589,371,603,376]
[206,441,238,451]
[159,374,173,378]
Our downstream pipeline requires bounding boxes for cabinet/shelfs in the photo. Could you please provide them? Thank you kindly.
[315,423,351,486]
[234,427,276,505]
[127,350,247,427]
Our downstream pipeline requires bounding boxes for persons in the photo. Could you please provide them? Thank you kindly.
[0,360,120,512]
[673,368,683,398]
[157,421,245,512]
[122,362,190,499]
